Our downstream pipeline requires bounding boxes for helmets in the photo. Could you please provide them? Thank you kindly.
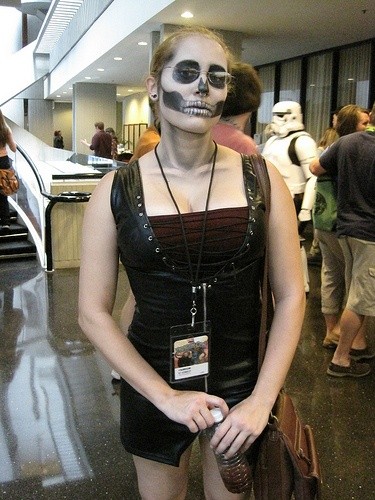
[270,101,304,138]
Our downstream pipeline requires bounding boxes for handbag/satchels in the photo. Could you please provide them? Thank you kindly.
[0,167,18,196]
[253,388,322,500]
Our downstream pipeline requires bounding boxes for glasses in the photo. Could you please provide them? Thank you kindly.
[158,65,232,86]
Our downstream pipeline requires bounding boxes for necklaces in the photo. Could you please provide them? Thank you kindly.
[220,117,241,128]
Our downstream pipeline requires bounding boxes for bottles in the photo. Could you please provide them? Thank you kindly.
[209,406,252,494]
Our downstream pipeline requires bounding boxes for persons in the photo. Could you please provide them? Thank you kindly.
[261,101,319,299]
[79,122,111,160]
[309,104,369,355]
[0,109,16,228]
[309,103,375,377]
[78,27,306,500]
[54,131,64,149]
[105,127,119,161]
[210,62,262,157]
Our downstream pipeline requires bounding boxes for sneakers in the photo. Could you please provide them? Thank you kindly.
[326,345,375,377]
[323,332,341,348]
